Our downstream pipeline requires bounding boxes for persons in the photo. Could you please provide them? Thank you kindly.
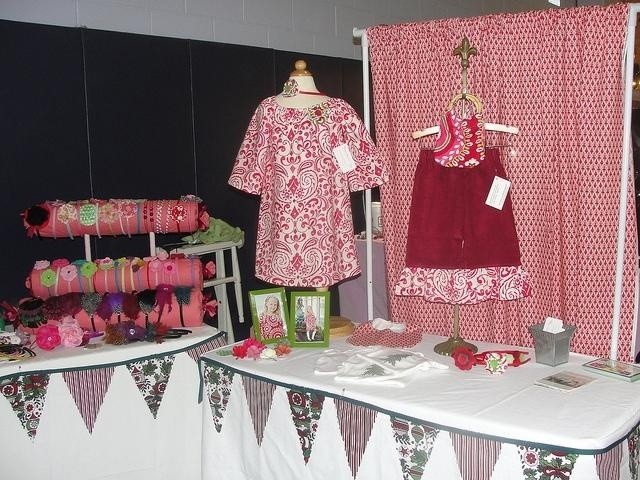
[259,296,284,340]
[292,295,321,343]
[233,63,393,288]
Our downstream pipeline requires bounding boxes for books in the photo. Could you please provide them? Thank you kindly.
[533,368,596,392]
[582,356,640,383]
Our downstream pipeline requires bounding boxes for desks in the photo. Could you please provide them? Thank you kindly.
[0,313,230,479]
[197,317,640,478]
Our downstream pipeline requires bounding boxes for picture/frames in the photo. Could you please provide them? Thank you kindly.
[248,286,332,348]
